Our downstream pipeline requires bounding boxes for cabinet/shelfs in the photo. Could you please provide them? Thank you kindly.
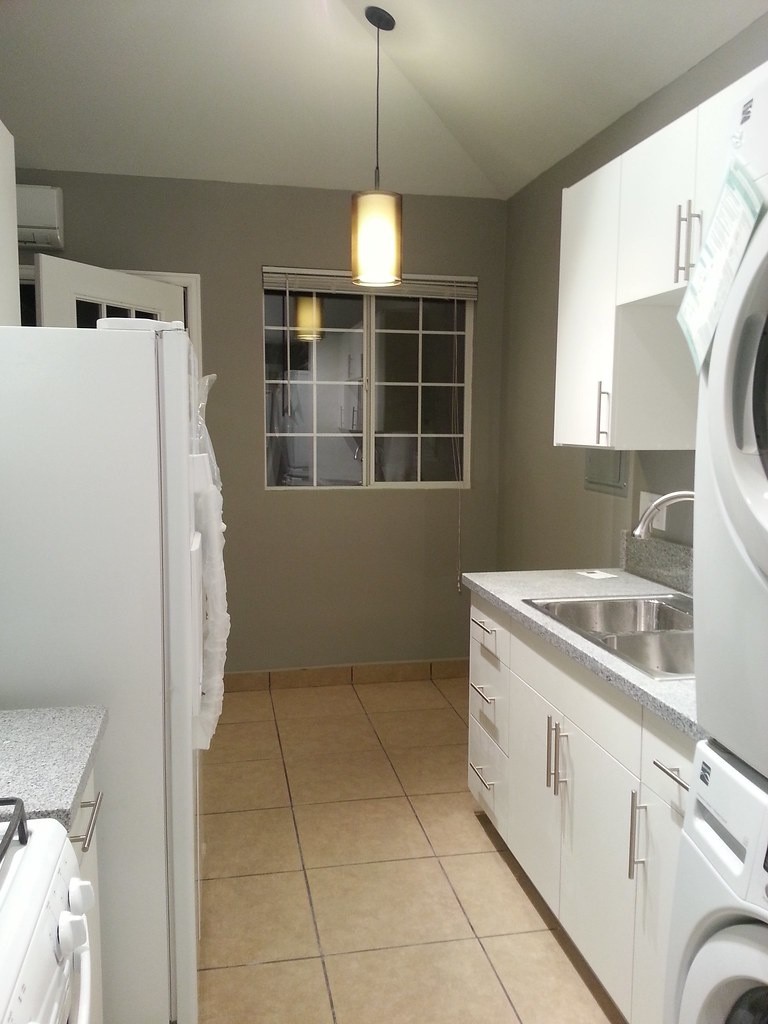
[468,594,700,1024]
[553,59,768,451]
[68,767,103,1024]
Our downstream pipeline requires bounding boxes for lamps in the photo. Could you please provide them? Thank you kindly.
[349,6,404,288]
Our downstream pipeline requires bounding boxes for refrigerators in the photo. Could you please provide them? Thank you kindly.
[0,318,211,1024]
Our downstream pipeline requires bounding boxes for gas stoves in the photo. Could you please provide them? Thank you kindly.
[0,796,99,1024]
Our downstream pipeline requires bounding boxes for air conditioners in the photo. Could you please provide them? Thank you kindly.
[16,183,65,250]
